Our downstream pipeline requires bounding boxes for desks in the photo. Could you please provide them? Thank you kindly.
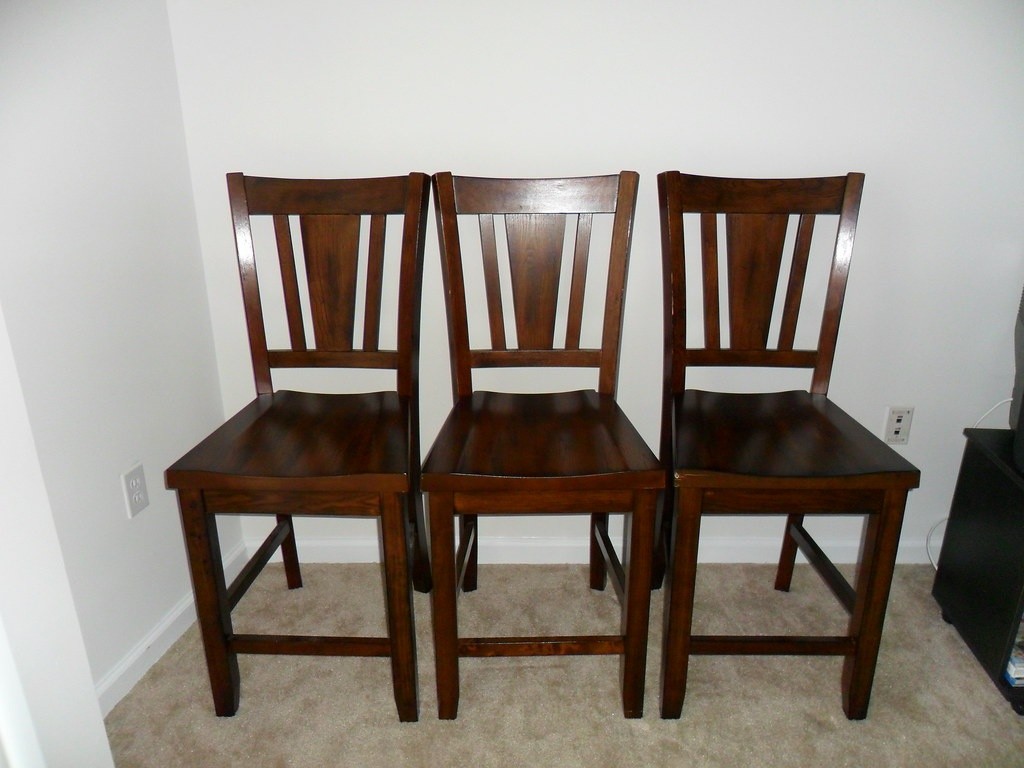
[931,427,1024,716]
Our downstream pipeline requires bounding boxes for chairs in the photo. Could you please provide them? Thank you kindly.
[656,170,921,719]
[422,170,664,718]
[164,171,429,721]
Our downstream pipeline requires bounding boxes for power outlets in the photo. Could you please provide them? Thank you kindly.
[124,463,148,519]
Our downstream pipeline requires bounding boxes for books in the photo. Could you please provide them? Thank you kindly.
[1004,623,1024,687]
